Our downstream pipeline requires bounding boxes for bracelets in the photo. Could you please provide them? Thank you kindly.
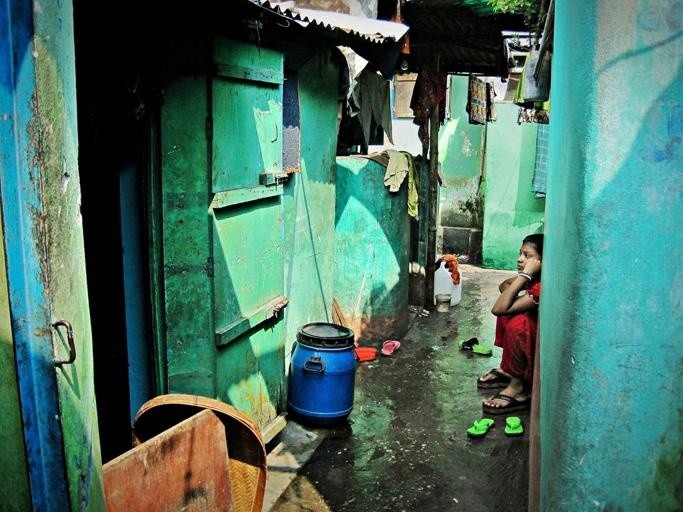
[518,271,534,281]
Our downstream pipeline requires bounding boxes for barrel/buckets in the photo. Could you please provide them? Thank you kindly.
[432,257,462,308]
[287,321,355,420]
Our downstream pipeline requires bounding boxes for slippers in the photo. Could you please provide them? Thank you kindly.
[458,337,492,356]
[504,416,524,435]
[380,340,401,356]
[466,417,495,436]
[477,369,529,414]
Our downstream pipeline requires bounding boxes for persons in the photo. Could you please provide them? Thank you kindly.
[477,234,543,414]
